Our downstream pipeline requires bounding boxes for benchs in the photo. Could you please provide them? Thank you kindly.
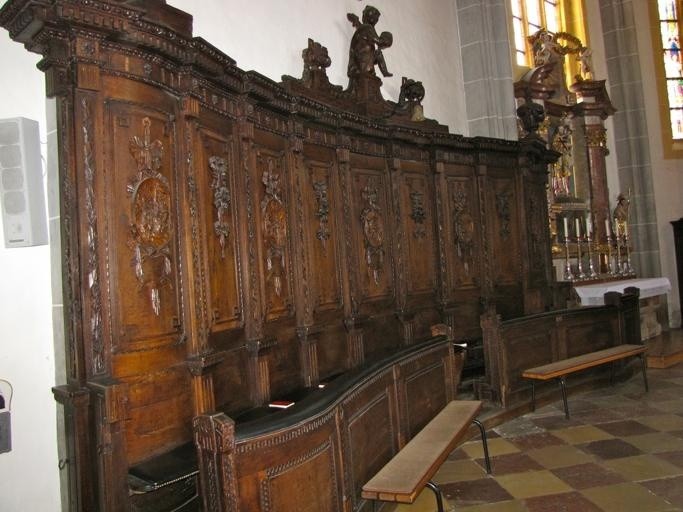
[453,338,485,359]
[515,338,657,422]
[121,372,345,491]
[356,395,494,511]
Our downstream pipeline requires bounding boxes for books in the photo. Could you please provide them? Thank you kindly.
[269,400,296,410]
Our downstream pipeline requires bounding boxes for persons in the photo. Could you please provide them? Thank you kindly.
[347,5,392,77]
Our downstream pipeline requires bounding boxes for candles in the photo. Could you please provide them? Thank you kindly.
[557,212,631,242]
